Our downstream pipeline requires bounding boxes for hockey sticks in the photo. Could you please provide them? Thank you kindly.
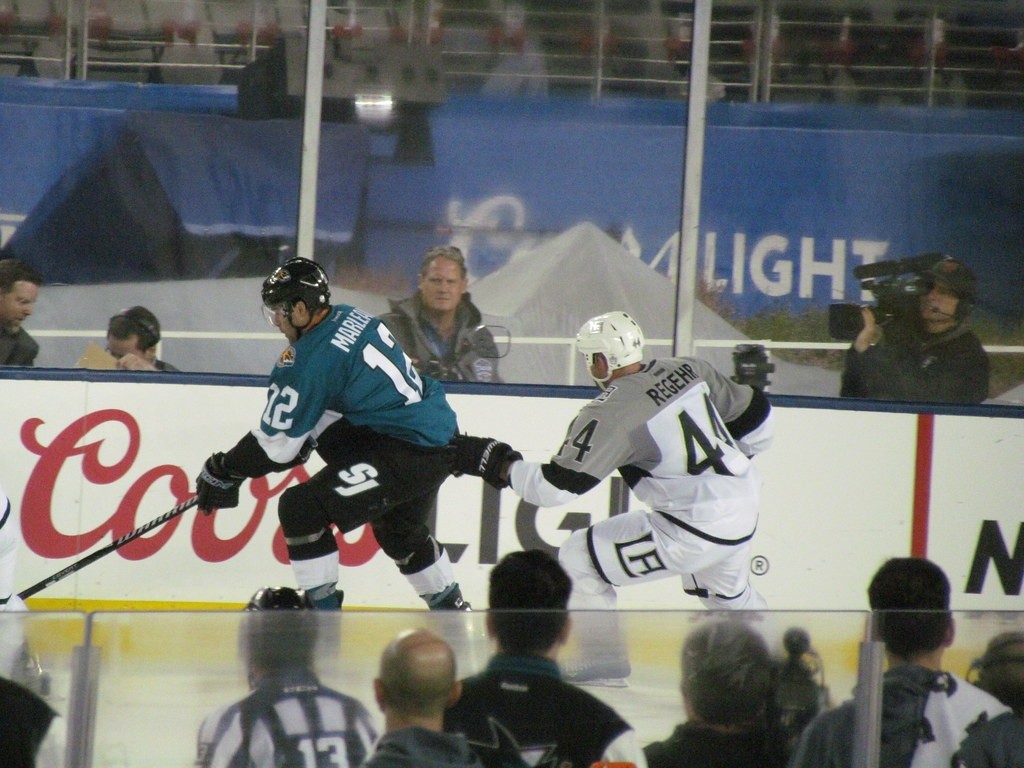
[0,491,208,611]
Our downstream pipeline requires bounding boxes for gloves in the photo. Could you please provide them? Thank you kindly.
[445,431,523,490]
[273,435,318,473]
[196,452,247,516]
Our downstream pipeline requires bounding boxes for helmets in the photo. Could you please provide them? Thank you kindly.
[236,587,317,661]
[576,311,644,370]
[261,257,331,311]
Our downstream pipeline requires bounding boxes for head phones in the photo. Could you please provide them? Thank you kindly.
[944,258,976,321]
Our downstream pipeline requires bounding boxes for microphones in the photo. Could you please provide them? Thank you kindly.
[932,307,957,319]
[854,260,900,279]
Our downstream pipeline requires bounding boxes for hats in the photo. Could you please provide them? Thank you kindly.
[919,260,980,299]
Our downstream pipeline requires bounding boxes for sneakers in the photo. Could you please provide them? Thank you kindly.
[560,658,630,686]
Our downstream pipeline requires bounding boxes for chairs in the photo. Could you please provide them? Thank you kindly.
[1,0,681,98]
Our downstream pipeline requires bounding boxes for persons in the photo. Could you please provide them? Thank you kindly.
[106,306,179,372]
[641,619,788,768]
[0,259,40,366]
[840,261,990,404]
[196,586,380,768]
[455,311,775,686]
[194,257,474,612]
[374,245,500,382]
[789,558,1014,768]
[975,630,1024,716]
[441,551,639,768]
[358,627,482,768]
